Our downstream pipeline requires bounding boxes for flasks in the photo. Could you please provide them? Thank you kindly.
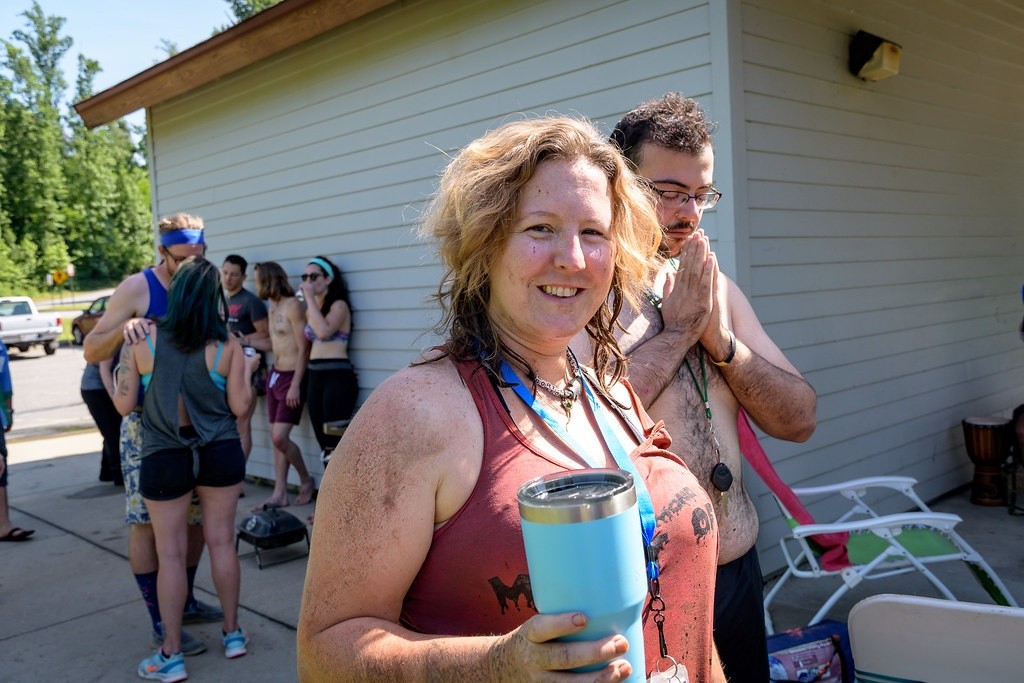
[516,467,649,683]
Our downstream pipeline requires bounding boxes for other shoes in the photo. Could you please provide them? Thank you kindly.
[179,599,224,622]
[152,622,207,656]
[99,469,111,480]
[112,477,124,486]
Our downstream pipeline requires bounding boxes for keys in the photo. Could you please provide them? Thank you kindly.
[711,462,734,517]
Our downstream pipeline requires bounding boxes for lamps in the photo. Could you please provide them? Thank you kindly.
[848,28,900,83]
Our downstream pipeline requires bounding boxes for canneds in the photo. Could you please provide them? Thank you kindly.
[295,288,305,301]
[243,347,256,357]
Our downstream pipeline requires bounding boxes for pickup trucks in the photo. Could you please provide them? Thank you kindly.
[0,297,63,355]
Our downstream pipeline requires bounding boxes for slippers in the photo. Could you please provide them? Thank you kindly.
[0,528,34,541]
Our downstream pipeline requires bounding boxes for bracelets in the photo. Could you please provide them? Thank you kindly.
[7,409,13,413]
[708,328,736,366]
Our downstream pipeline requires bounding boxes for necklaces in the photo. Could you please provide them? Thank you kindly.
[498,340,582,414]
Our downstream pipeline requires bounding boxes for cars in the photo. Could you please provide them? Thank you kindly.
[72,295,111,344]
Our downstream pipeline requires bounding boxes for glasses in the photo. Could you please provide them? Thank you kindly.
[255,262,261,271]
[643,180,722,208]
[164,246,184,265]
[302,272,326,281]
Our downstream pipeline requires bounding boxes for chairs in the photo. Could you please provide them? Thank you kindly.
[14,305,26,314]
[849,593,1024,682]
[735,407,1019,633]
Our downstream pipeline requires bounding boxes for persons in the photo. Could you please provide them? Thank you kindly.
[85,213,226,658]
[81,255,362,524]
[604,91,818,682]
[99,253,261,683]
[295,115,729,683]
[0,337,36,542]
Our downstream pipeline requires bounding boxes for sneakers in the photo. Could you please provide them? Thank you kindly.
[221,628,250,658]
[138,645,188,683]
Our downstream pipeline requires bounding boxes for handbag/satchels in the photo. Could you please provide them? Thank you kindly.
[766,619,856,683]
